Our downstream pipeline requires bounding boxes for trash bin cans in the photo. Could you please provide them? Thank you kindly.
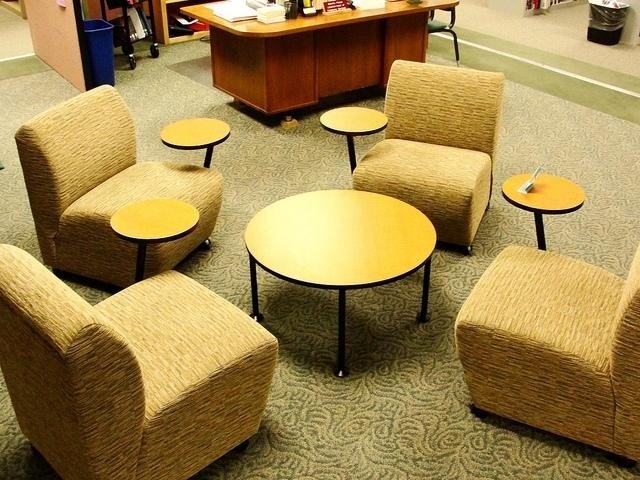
[81,18,115,90]
[588,0,631,47]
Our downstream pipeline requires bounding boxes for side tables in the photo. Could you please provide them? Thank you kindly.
[501,174,585,250]
[161,117,230,168]
[319,106,388,173]
[109,197,199,282]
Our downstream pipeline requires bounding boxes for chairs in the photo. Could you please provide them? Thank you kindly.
[351,60,504,254]
[455,245,640,467]
[427,6,459,61]
[15,84,223,289]
[0,244,279,480]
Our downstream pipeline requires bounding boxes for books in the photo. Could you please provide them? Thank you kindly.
[213,4,258,23]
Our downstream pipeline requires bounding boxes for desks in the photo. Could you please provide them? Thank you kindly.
[179,0,460,129]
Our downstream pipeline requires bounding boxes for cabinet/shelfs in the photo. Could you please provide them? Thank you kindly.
[152,0,210,44]
